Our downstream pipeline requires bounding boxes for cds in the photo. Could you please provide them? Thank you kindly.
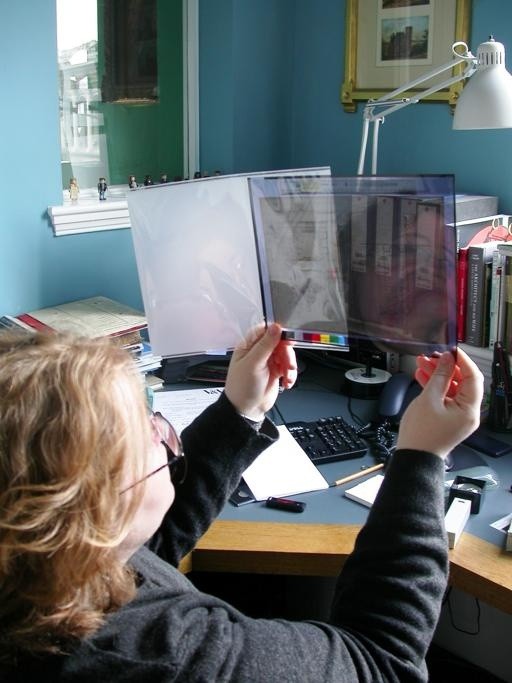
[344,367,393,400]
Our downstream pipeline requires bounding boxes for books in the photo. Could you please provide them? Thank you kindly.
[458,242,512,433]
[0,291,170,393]
[338,191,498,345]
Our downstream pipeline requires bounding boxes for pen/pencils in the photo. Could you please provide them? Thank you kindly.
[487,342,511,432]
[328,463,384,487]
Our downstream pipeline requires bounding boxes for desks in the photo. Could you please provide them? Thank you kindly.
[150,378,512,614]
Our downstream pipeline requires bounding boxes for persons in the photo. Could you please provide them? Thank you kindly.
[0,317,487,683]
[64,168,220,202]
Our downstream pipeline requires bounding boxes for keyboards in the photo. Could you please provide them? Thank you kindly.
[286,414,368,465]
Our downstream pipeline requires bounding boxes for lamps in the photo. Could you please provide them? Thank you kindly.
[354,35,512,192]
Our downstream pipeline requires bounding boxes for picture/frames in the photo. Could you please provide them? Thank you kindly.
[340,0,472,114]
[47,0,200,236]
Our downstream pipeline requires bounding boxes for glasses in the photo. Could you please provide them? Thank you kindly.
[114,400,188,502]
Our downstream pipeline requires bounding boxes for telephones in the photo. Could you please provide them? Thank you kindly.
[377,373,423,430]
